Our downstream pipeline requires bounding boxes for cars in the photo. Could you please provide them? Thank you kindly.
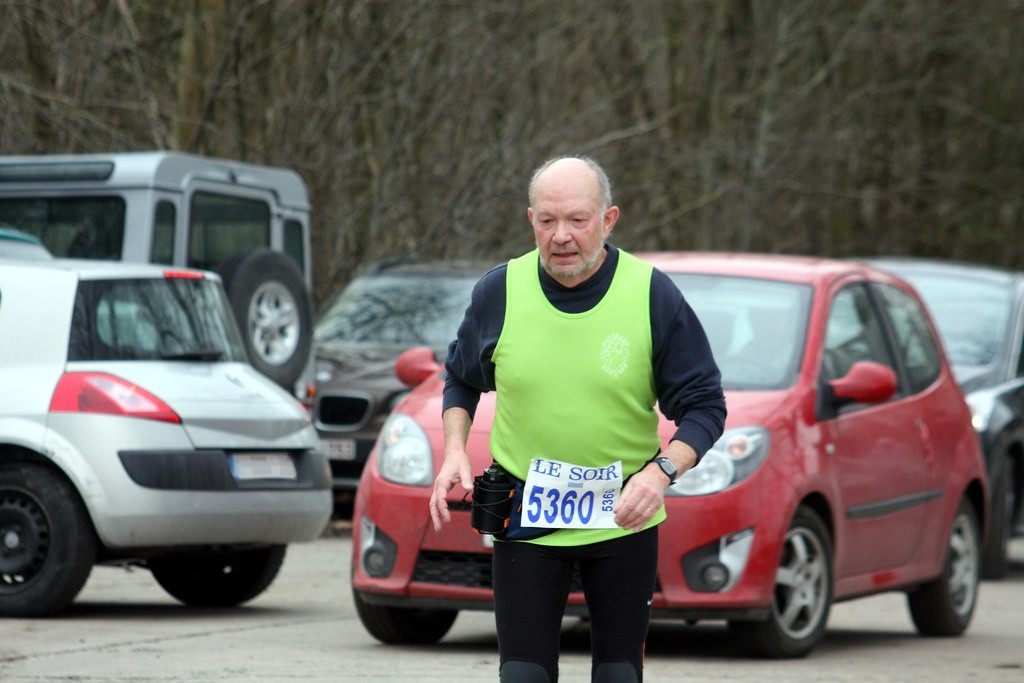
[349,251,990,660]
[826,257,1023,583]
[0,256,334,618]
[296,259,503,522]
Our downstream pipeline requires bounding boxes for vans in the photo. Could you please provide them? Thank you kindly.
[0,153,317,413]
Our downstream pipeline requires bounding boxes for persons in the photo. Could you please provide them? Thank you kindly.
[427,155,728,683]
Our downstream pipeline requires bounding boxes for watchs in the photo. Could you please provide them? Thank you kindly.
[654,457,677,485]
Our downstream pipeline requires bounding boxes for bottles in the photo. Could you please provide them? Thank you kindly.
[483,468,503,484]
